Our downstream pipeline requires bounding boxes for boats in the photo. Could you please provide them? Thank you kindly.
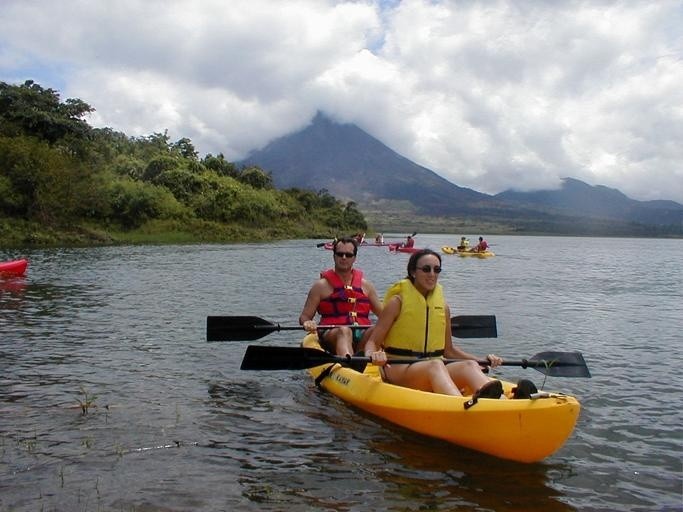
[300,331,581,463]
[325,242,495,258]
[0,259,27,276]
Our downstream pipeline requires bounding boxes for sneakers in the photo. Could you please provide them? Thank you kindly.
[471,380,503,400]
[511,380,538,401]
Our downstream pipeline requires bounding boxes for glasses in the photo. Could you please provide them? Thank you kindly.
[416,265,443,274]
[332,251,355,259]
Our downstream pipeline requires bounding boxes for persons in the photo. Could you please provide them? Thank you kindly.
[329,231,385,247]
[403,235,414,248]
[456,237,468,250]
[467,236,488,253]
[362,247,537,398]
[296,234,385,358]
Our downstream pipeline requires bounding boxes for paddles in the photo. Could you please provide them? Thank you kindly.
[207,315,497,341]
[316,234,359,248]
[395,232,417,251]
[240,345,590,377]
[457,244,495,249]
